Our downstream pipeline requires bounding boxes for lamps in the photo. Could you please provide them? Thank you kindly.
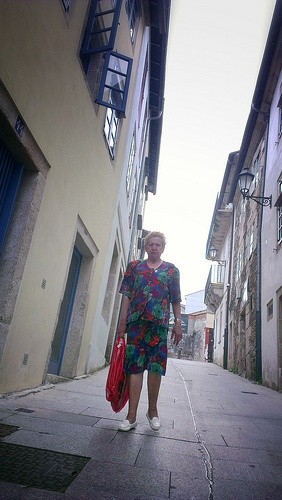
[236,168,272,209]
[208,246,226,267]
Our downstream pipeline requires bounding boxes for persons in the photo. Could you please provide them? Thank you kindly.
[116,232,182,431]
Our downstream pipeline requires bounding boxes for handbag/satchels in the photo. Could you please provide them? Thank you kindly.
[105,337,130,415]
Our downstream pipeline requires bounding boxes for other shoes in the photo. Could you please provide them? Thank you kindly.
[146,410,162,431]
[118,419,137,431]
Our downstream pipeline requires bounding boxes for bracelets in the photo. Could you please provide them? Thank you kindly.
[173,319,182,323]
